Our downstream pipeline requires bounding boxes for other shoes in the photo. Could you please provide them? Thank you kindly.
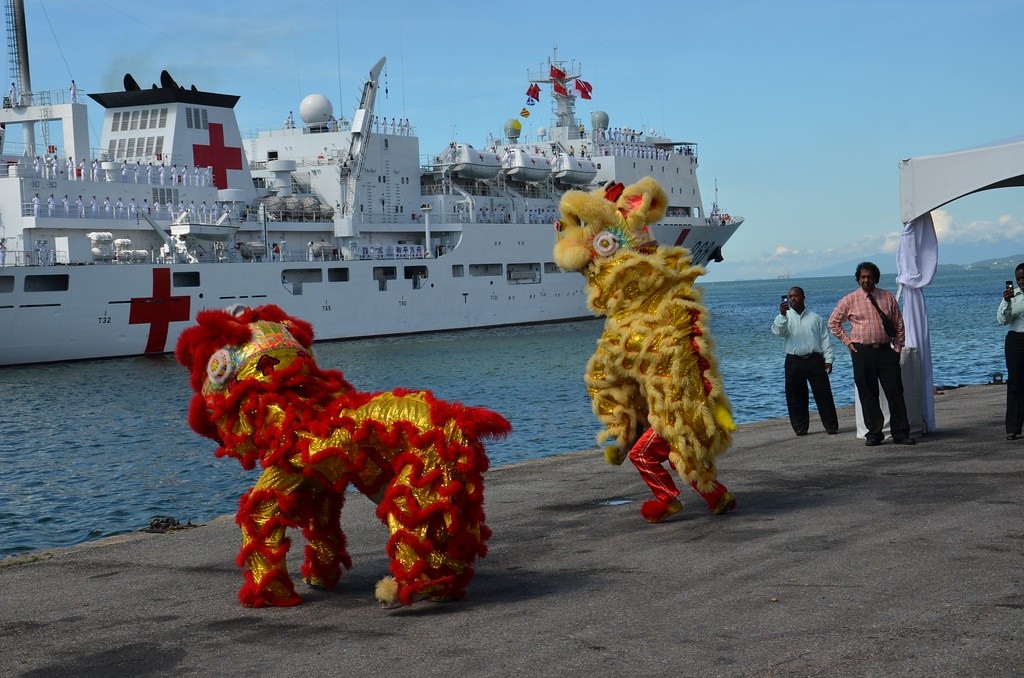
[866,438,883,446]
[1006,432,1016,440]
[381,590,434,611]
[893,438,916,445]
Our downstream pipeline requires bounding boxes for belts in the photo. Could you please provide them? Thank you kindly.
[855,343,891,348]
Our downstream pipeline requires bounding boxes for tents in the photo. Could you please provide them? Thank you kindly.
[896,133,1024,437]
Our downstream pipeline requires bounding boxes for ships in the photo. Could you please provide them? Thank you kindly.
[0,0,745,368]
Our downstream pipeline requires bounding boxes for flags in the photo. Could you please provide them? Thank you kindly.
[519,107,530,118]
[512,119,521,129]
[575,79,592,100]
[526,96,535,106]
[526,83,540,102]
[551,65,567,96]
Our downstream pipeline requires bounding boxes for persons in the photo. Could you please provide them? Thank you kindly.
[997,263,1024,441]
[10,82,17,108]
[70,80,76,104]
[712,204,719,214]
[288,111,296,128]
[828,261,917,446]
[459,124,688,224]
[374,116,409,136]
[0,154,280,267]
[771,286,839,438]
[327,115,335,133]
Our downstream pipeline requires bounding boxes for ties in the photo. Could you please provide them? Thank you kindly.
[867,293,898,337]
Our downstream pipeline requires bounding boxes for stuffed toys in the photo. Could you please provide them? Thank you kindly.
[553,175,737,522]
[175,304,513,609]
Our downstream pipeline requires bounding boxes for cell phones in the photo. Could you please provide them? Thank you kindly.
[781,295,790,311]
[1006,281,1014,298]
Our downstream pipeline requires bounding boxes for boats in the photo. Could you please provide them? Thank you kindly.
[169,223,240,243]
[501,148,551,181]
[551,153,597,183]
[432,143,502,180]
